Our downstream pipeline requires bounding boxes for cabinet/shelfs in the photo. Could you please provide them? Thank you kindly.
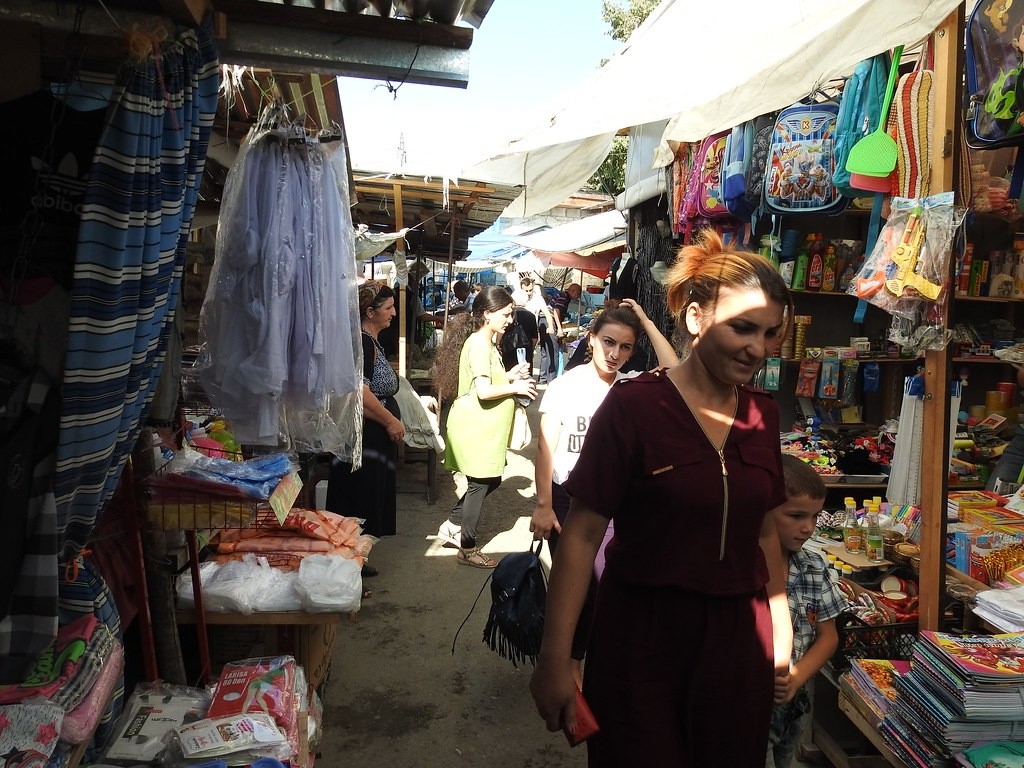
[624,0,1024,768]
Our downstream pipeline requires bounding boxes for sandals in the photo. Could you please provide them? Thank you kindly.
[457,547,498,569]
[438,522,461,549]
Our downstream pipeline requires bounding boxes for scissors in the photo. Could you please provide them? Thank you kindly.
[822,366,837,397]
[767,368,778,388]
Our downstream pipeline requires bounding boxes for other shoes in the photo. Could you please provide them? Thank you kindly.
[361,563,378,576]
[539,381,547,385]
[362,586,371,598]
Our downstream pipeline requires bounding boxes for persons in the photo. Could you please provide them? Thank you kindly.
[529,298,679,691]
[435,274,484,316]
[547,292,553,302]
[422,277,441,307]
[537,284,582,384]
[326,280,405,598]
[520,277,554,351]
[763,454,849,768]
[429,285,538,568]
[528,228,796,768]
[242,665,285,713]
[377,260,445,361]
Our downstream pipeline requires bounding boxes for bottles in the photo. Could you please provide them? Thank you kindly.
[208,420,241,462]
[841,497,861,554]
[861,496,885,563]
[186,427,228,460]
[792,232,864,292]
[826,554,852,580]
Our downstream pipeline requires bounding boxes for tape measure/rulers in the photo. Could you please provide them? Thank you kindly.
[904,394,926,507]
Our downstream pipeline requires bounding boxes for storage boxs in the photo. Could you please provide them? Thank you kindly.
[206,622,336,692]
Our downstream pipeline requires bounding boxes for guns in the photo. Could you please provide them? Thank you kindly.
[885,206,942,300]
[858,219,901,299]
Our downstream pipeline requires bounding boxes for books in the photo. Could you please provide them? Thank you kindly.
[843,631,1024,768]
[956,244,994,296]
[947,490,1024,524]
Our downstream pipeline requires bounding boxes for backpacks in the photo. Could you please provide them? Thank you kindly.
[963,0,1024,199]
[698,101,850,262]
[831,50,899,198]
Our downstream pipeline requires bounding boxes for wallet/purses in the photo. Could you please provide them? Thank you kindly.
[561,682,599,749]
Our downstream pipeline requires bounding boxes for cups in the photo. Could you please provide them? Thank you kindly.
[971,381,1018,423]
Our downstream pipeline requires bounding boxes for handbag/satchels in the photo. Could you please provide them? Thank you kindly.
[480,536,549,668]
[499,308,529,356]
[507,405,532,450]
